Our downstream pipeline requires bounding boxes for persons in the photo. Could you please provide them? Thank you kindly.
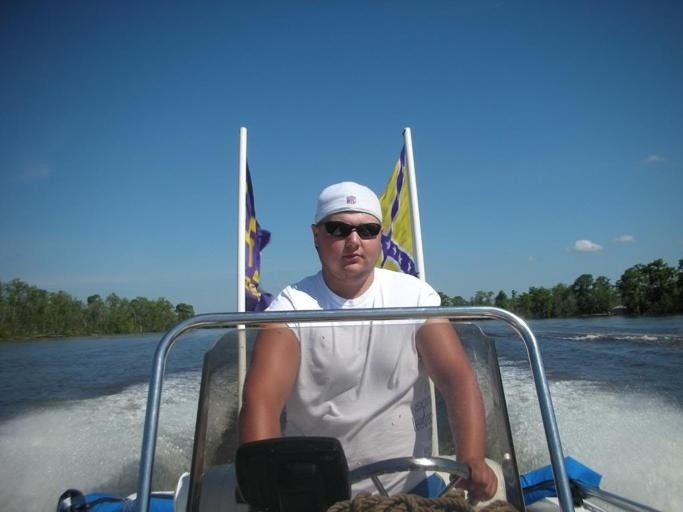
[240,179,499,505]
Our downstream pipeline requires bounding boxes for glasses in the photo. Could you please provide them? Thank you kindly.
[320,219,384,237]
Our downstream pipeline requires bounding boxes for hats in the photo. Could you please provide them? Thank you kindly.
[314,179,383,223]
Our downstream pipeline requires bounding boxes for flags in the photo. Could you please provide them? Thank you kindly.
[373,142,428,280]
[244,154,275,324]
